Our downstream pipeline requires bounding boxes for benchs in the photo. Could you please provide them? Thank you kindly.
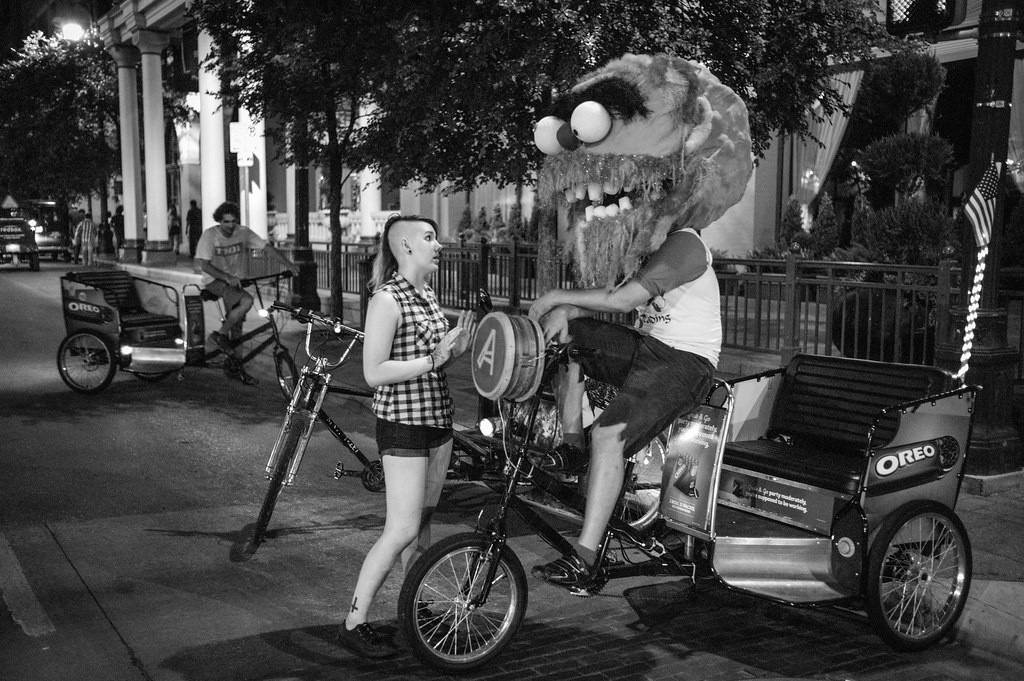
[722,355,962,495]
[67,271,179,329]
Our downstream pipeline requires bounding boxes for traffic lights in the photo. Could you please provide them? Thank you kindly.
[886,0,967,36]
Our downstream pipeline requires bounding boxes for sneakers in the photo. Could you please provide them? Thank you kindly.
[417,602,455,636]
[531,556,592,592]
[208,331,235,356]
[242,373,259,386]
[529,443,590,476]
[337,619,396,659]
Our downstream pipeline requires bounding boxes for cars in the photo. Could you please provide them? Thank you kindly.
[0,215,40,271]
[15,207,72,262]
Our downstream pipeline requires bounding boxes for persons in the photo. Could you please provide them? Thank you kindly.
[527,224,722,597]
[194,202,300,386]
[185,200,203,256]
[11,201,183,267]
[334,215,480,659]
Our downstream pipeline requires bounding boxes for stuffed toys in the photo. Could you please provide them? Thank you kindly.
[530,52,754,289]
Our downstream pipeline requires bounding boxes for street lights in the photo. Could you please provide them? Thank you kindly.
[61,2,116,258]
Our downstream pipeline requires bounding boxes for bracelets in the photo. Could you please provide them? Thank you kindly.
[429,352,435,372]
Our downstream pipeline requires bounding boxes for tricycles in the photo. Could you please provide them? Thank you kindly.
[253,302,671,538]
[396,289,981,672]
[56,270,300,403]
